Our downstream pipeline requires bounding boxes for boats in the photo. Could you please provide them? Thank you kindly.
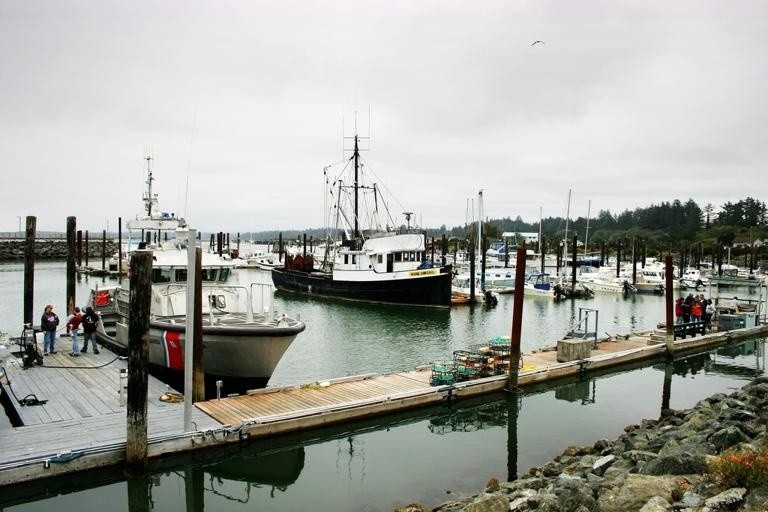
[172,446,306,505]
[272,136,454,313]
[84,280,304,400]
[426,187,768,304]
[209,231,340,272]
[108,153,236,283]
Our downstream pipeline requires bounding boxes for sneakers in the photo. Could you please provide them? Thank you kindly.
[81,349,87,353]
[75,353,80,356]
[50,349,57,354]
[68,351,74,357]
[43,349,48,355]
[94,350,100,354]
[69,332,74,337]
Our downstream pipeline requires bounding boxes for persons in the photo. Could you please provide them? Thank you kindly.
[730,296,742,314]
[66,306,83,357]
[675,293,717,334]
[691,355,704,375]
[41,304,59,355]
[81,307,101,355]
[426,255,432,262]
[66,311,78,337]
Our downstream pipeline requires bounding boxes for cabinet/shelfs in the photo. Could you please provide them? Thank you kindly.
[429,336,524,388]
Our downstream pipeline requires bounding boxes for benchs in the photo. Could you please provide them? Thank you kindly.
[674,320,707,340]
